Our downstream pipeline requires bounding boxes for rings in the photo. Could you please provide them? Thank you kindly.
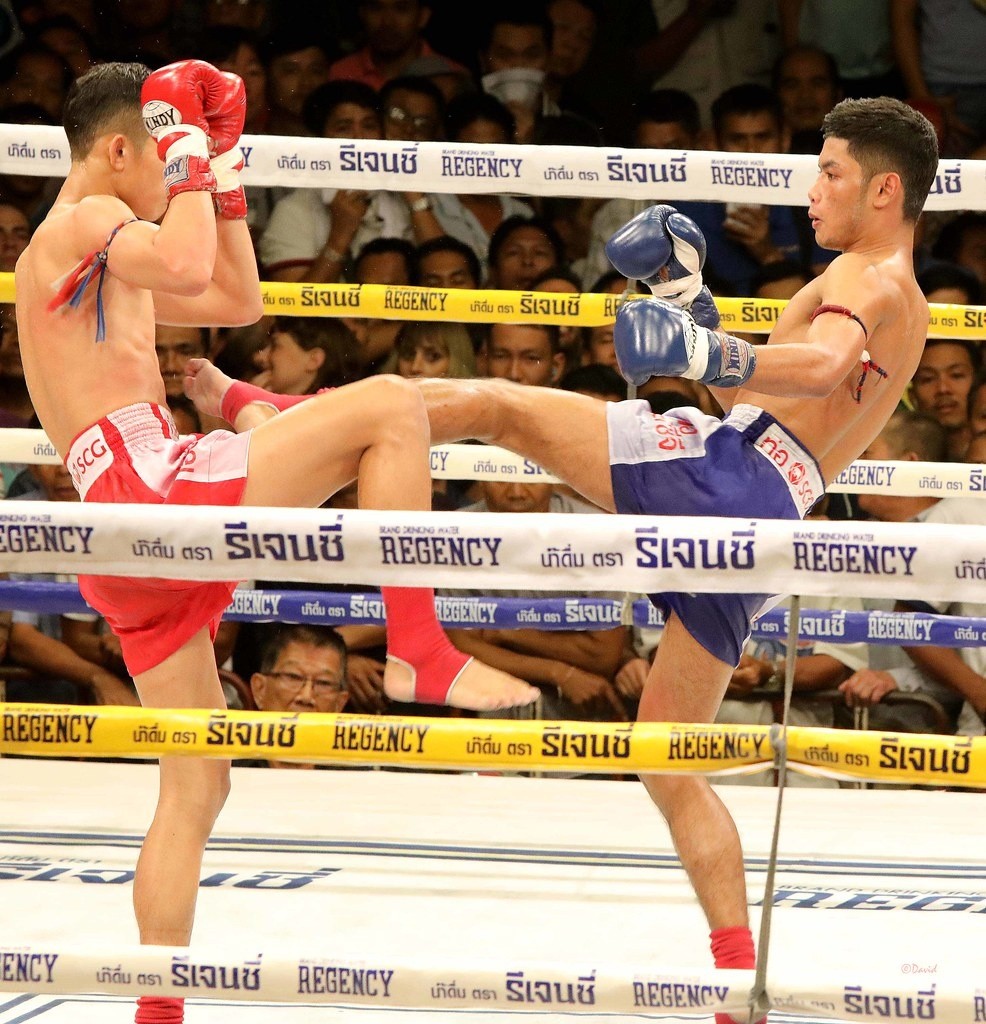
[362,198,371,205]
[368,671,378,681]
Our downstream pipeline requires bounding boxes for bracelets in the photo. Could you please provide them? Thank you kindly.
[410,197,434,212]
[557,667,576,697]
[322,246,344,264]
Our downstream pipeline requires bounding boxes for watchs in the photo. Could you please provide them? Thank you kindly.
[765,663,782,692]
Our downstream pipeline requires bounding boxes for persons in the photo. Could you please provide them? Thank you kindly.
[181,98,942,1024]
[14,58,539,1024]
[0,0,985,794]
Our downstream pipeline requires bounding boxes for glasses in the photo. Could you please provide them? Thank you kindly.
[386,106,434,131]
[262,669,344,700]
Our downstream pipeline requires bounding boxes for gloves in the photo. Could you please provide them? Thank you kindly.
[203,71,248,220]
[139,59,228,202]
[606,203,721,329]
[615,297,756,388]
